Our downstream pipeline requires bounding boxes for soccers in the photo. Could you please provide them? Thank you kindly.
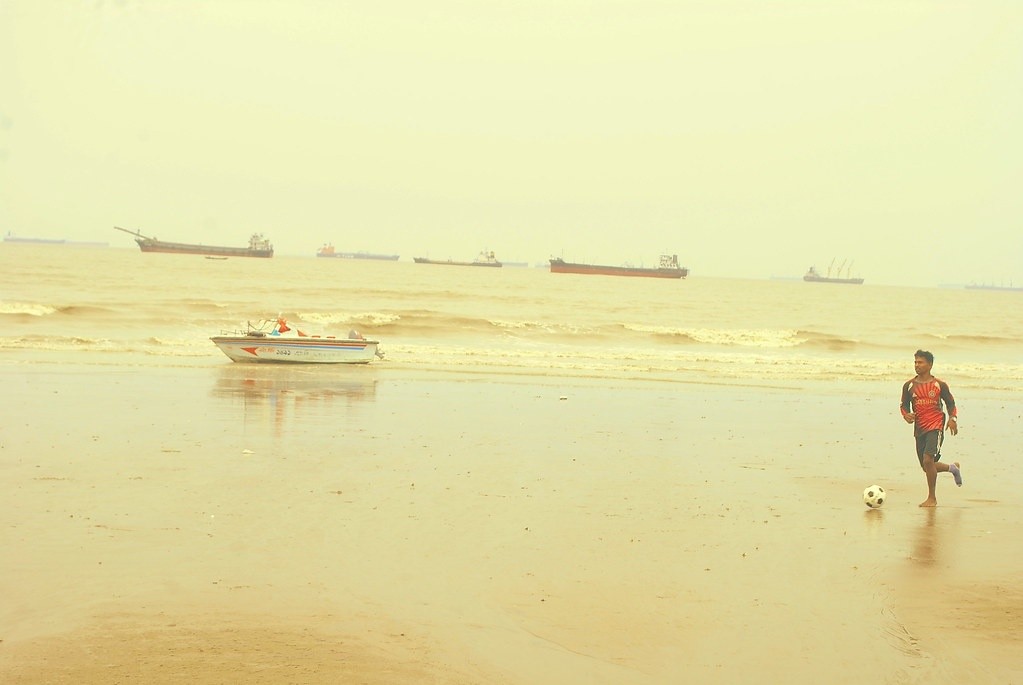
[863,484,886,508]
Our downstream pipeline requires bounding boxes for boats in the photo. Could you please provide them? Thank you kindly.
[549,246,688,278]
[208,314,386,364]
[413,251,503,268]
[803,258,864,284]
[115,226,274,257]
[316,242,399,262]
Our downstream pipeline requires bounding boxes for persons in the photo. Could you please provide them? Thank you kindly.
[900,350,962,507]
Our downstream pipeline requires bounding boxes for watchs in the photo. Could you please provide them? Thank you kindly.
[950,416,957,422]
